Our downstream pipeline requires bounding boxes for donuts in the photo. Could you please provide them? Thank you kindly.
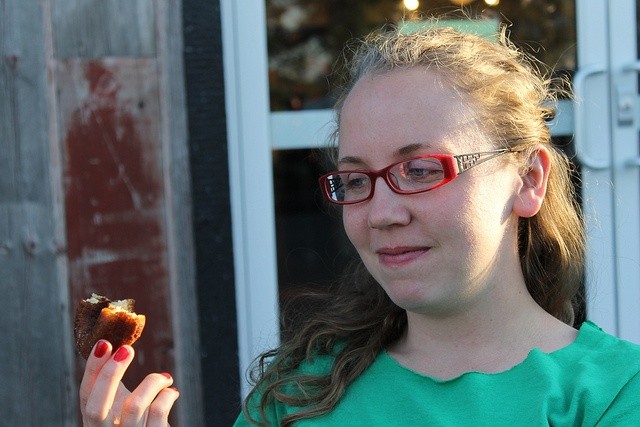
[74,294,146,360]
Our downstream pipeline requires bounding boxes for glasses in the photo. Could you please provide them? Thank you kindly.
[319,148,527,205]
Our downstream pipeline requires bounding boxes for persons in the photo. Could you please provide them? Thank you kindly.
[80,6,640,426]
